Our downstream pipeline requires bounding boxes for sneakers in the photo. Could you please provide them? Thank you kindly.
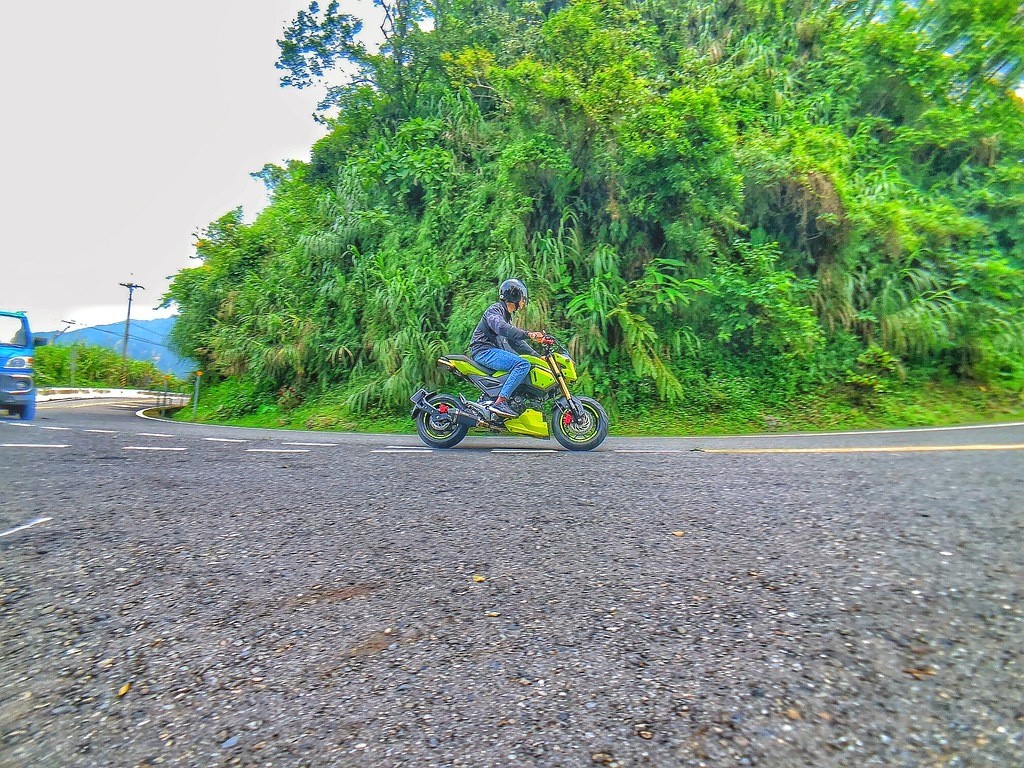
[489,400,519,418]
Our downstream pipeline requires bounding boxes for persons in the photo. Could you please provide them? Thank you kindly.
[469,278,545,418]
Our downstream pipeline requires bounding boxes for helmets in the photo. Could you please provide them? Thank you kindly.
[499,279,527,303]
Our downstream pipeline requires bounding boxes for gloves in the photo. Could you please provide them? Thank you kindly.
[541,355,547,361]
[528,331,543,342]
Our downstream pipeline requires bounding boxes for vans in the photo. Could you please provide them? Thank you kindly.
[0,310,37,422]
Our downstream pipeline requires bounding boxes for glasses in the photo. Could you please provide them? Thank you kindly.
[521,295,526,302]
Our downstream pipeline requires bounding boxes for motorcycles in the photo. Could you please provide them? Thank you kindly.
[410,331,611,453]
